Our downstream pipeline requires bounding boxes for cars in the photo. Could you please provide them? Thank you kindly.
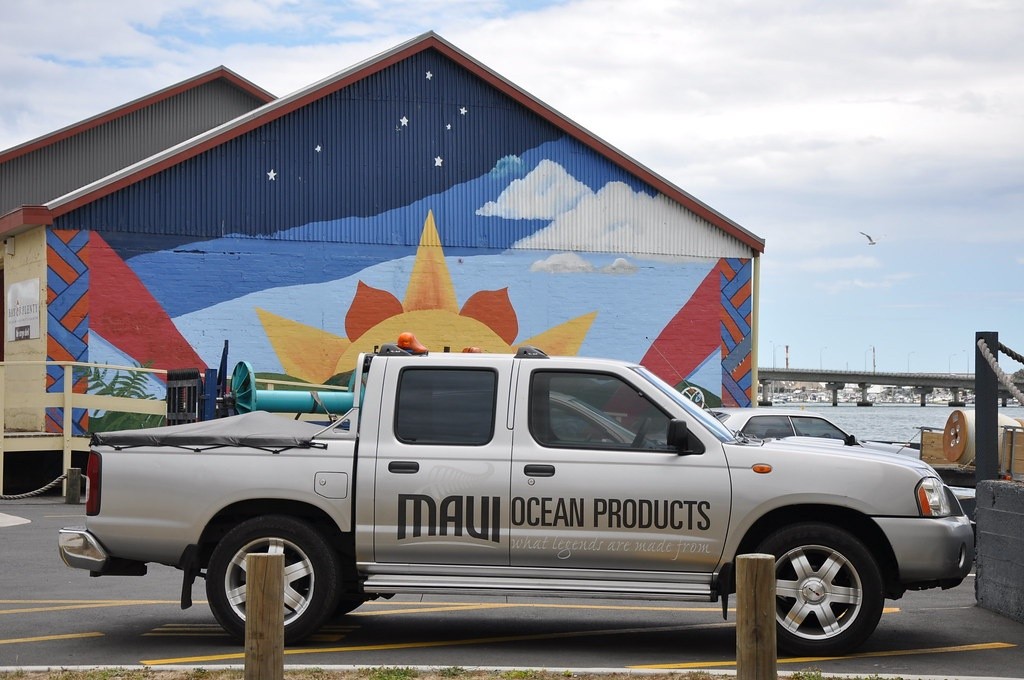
[755,382,975,405]
[410,387,665,451]
[651,407,922,461]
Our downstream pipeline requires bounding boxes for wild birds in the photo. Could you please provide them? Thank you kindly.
[859,232,885,245]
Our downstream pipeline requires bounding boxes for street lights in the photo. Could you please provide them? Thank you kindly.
[769,340,774,368]
[949,354,956,374]
[819,346,826,371]
[962,349,969,373]
[907,351,916,373]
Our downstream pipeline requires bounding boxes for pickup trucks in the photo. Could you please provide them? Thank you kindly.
[58,333,972,659]
